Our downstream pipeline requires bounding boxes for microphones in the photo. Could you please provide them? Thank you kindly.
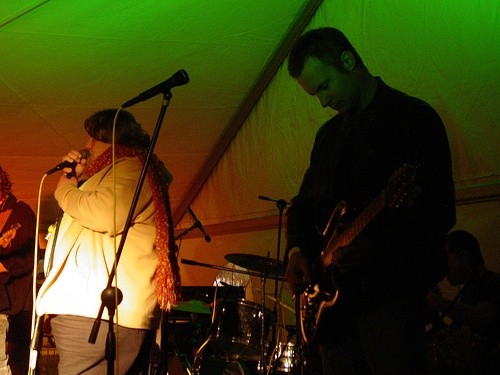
[122,70,191,109]
[186,207,211,243]
[46,149,90,175]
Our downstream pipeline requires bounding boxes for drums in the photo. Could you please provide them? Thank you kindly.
[263,340,302,375]
[198,356,263,375]
[207,298,274,362]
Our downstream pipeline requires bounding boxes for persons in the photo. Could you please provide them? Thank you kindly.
[283,27,457,375]
[35,108,176,375]
[428,230,500,375]
[0,165,40,375]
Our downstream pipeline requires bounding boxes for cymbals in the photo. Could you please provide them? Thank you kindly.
[224,253,286,276]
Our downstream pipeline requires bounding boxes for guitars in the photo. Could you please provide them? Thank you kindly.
[0,224,22,248]
[300,163,419,344]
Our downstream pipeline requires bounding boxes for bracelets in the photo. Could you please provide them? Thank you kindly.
[288,247,301,260]
